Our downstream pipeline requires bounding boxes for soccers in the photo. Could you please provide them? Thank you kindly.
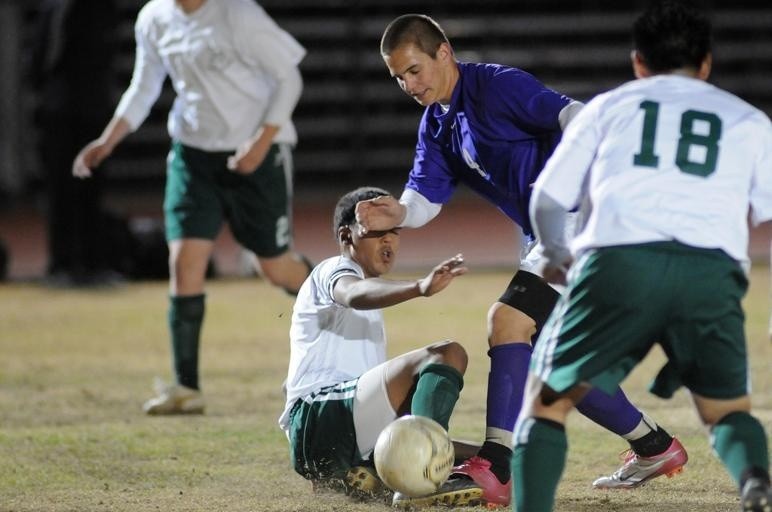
[373,414,456,496]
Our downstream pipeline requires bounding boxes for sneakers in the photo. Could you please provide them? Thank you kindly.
[741,467,772,512]
[342,455,512,511]
[593,438,688,488]
[143,385,205,414]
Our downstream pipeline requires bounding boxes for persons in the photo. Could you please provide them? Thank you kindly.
[280,188,483,510]
[70,1,317,415]
[356,14,687,507]
[510,7,772,512]
